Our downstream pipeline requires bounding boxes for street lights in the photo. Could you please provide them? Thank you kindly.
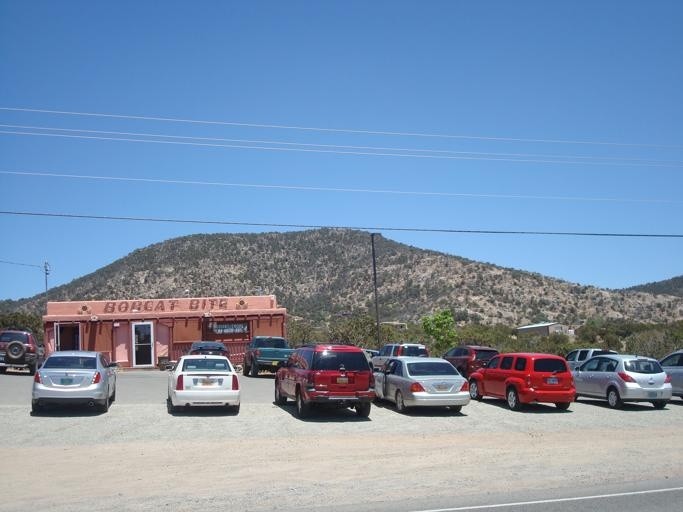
[371,232,382,353]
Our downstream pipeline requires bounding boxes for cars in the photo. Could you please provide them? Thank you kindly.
[374,355,471,413]
[168,355,241,412]
[188,341,229,359]
[571,353,673,409]
[659,349,683,396]
[31,350,117,412]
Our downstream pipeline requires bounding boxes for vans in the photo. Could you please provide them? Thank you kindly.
[469,352,578,410]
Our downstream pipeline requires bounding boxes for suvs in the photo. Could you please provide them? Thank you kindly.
[274,345,376,418]
[0,329,45,376]
[441,344,500,377]
[368,342,430,373]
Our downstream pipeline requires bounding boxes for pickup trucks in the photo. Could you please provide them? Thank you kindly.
[565,348,619,371]
[243,335,295,375]
